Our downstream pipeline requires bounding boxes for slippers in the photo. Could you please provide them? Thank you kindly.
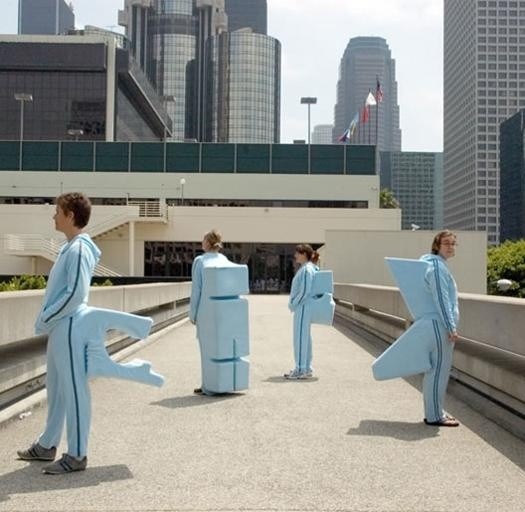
[424,416,455,420]
[425,420,459,426]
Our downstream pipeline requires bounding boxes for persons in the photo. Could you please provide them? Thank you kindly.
[419,230,459,426]
[17,193,101,474]
[284,245,320,379]
[189,229,228,395]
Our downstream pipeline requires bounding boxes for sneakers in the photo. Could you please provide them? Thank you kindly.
[17,440,57,460]
[289,368,311,375]
[194,388,203,396]
[43,453,88,474]
[284,373,313,379]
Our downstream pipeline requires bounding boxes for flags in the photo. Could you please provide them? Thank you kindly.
[339,80,383,144]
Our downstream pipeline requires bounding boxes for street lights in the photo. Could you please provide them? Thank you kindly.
[300,97,316,175]
[180,178,185,205]
[159,95,175,173]
[13,92,32,171]
[67,130,82,142]
